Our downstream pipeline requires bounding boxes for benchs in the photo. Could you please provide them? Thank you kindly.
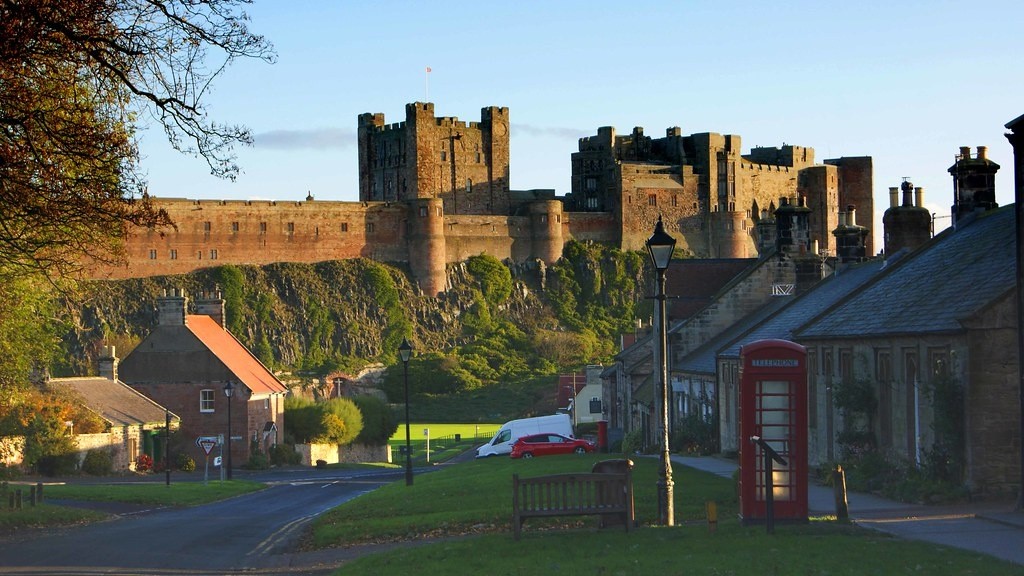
[512,467,633,540]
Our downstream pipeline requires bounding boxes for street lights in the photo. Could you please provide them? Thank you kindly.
[645,213,677,528]
[397,336,412,484]
[223,378,236,480]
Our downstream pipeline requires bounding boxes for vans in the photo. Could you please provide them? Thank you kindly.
[475,414,572,459]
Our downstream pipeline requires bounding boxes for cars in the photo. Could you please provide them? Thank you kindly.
[509,433,596,459]
